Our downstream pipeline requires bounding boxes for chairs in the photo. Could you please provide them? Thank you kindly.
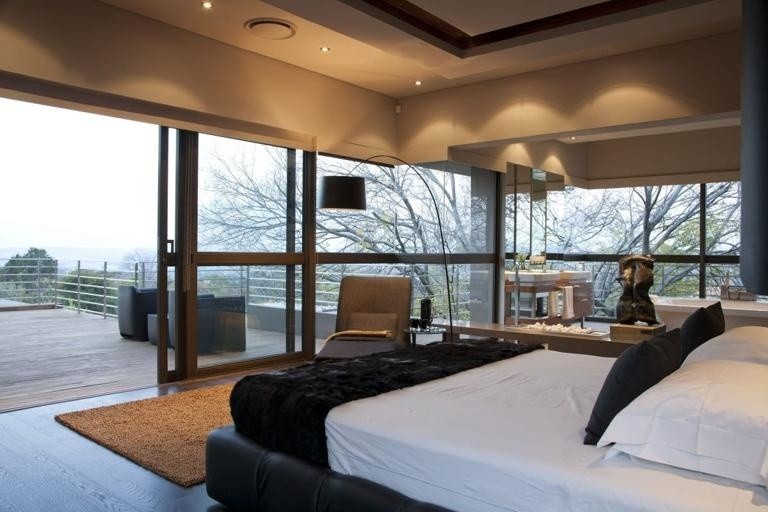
[315,275,413,360]
[117,284,247,354]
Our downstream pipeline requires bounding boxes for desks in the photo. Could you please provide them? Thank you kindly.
[505,269,593,330]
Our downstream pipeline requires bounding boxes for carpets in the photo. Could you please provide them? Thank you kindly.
[50,379,239,487]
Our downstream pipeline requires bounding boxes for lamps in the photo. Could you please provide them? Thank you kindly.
[317,156,454,338]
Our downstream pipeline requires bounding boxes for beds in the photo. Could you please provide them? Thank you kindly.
[204,338,768,512]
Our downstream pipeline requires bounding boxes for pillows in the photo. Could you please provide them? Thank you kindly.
[582,298,768,488]
